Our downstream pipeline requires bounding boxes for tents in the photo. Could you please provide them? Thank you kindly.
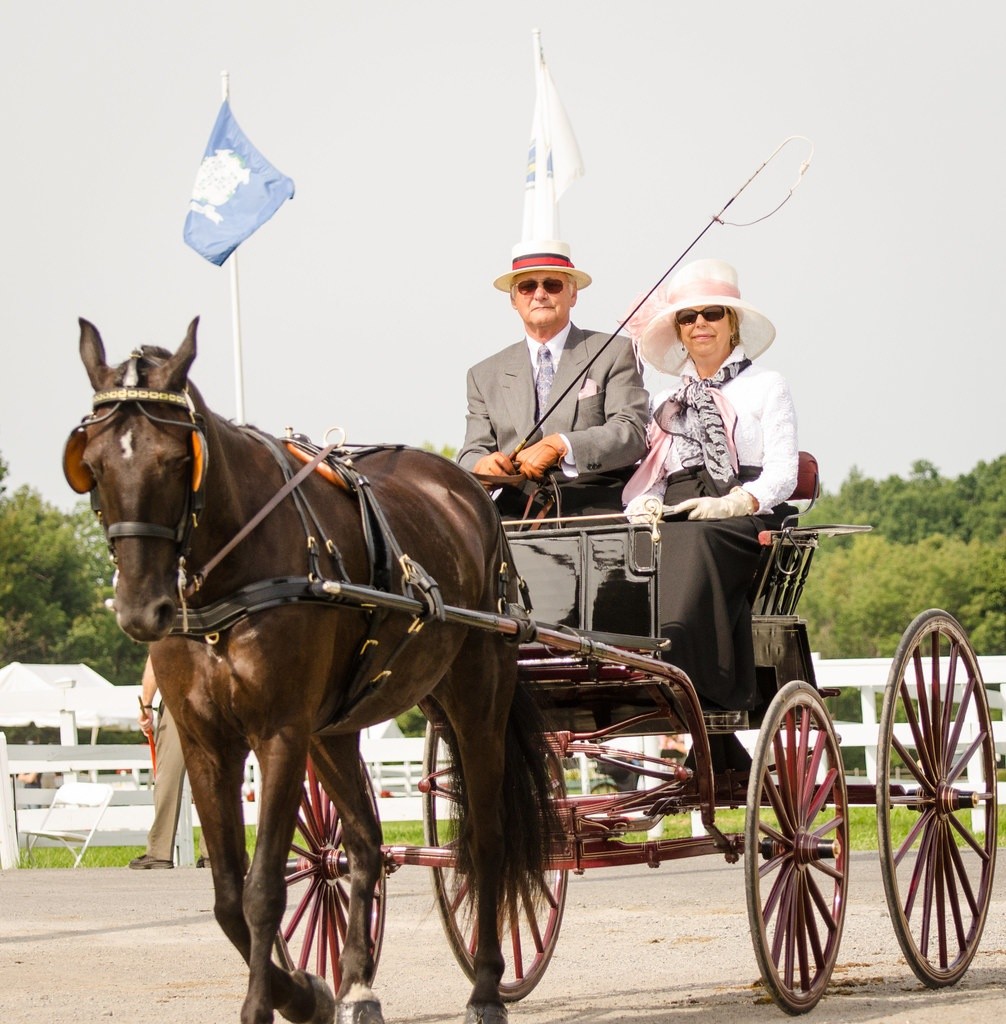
[0,661,161,744]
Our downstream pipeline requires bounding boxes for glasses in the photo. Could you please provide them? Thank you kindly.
[511,280,574,296]
[674,305,728,325]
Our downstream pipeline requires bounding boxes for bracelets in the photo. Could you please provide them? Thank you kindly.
[143,705,152,708]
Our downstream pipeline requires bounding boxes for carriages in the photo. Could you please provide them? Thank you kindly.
[59,316,1000,1023]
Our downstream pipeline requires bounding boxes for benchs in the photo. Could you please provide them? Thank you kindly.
[419,452,820,734]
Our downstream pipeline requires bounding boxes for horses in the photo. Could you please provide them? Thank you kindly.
[75,312,576,1024]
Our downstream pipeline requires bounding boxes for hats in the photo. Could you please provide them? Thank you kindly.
[616,258,776,377]
[493,240,592,293]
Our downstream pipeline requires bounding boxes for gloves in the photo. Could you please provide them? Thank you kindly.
[624,495,671,524]
[515,433,569,481]
[673,487,754,520]
[473,452,517,491]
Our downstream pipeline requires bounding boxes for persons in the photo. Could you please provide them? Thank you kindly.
[457,239,650,528]
[130,656,211,869]
[621,259,799,694]
[19,737,42,809]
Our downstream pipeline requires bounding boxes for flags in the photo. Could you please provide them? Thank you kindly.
[521,61,584,239]
[183,102,294,266]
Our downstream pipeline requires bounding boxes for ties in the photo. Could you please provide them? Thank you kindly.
[536,344,557,430]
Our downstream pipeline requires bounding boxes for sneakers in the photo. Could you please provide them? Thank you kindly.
[129,854,173,870]
[196,857,211,867]
